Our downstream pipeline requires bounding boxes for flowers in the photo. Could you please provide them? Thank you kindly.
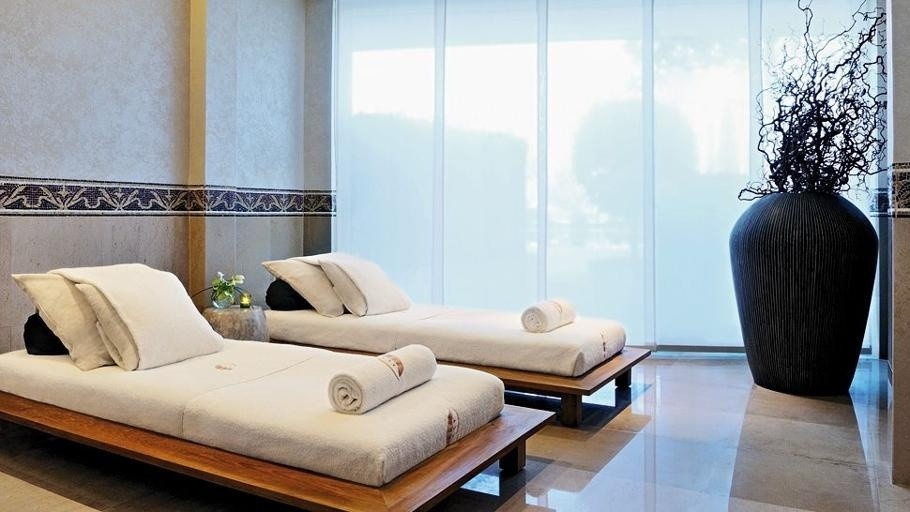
[192,265,253,308]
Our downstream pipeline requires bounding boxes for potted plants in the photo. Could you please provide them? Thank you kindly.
[728,0,887,399]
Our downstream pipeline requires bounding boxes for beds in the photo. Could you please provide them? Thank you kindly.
[262,302,651,427]
[1,337,563,512]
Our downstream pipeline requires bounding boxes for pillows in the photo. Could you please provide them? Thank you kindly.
[260,252,410,316]
[10,262,222,371]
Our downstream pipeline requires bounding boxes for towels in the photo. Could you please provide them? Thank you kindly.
[521,298,574,332]
[329,341,437,414]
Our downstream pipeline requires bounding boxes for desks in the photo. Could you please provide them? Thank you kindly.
[200,306,268,340]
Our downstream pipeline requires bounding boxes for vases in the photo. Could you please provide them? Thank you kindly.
[211,290,234,308]
